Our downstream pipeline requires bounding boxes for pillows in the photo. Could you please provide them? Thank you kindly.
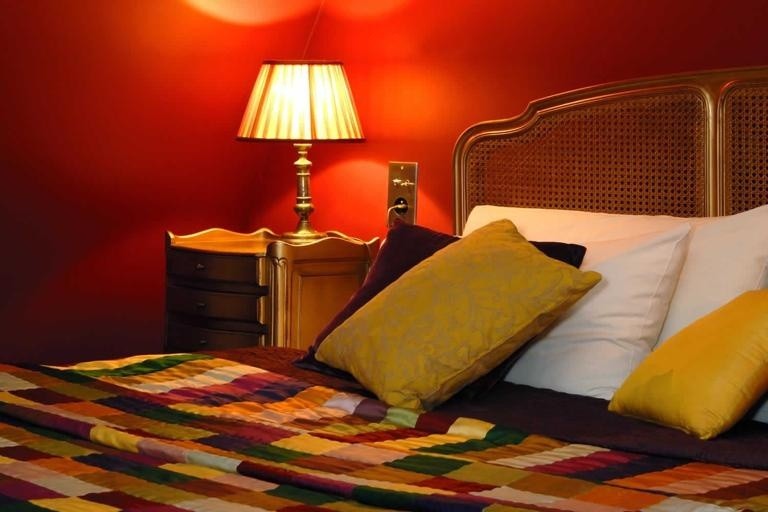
[314,218,602,412]
[293,225,587,403]
[608,289,767,442]
[461,204,767,352]
[503,227,692,400]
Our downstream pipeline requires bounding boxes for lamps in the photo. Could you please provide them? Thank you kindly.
[234,59,367,239]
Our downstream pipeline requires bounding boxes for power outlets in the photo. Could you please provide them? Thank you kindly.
[387,161,418,229]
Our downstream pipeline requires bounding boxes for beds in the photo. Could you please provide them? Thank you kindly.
[0,65,767,512]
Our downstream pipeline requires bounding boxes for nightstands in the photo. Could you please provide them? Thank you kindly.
[164,229,380,359]
